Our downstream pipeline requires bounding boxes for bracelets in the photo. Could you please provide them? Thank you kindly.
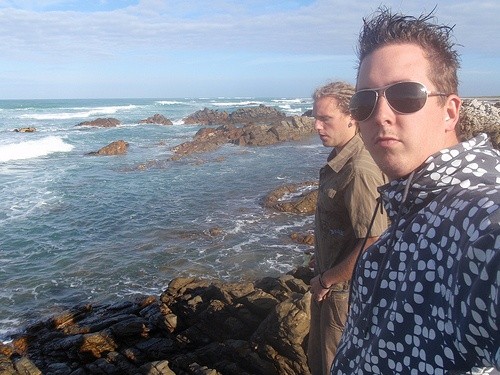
[319,273,334,289]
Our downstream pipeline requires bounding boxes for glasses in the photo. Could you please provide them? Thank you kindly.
[348,81,450,122]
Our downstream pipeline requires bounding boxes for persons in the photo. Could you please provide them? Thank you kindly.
[306,81,390,375]
[331,5,500,375]
